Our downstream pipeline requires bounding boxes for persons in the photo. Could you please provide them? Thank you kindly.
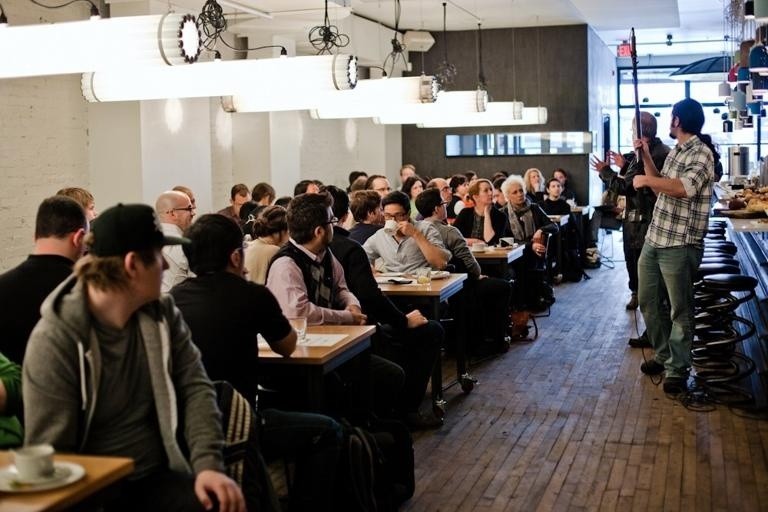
[589,112,670,349]
[0,194,85,367]
[0,352,23,452]
[56,155,641,512]
[700,133,723,183]
[637,99,715,394]
[20,204,246,512]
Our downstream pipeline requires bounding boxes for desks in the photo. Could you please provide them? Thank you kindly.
[371,270,472,428]
[547,211,570,282]
[572,205,592,283]
[257,320,378,412]
[0,449,136,512]
[460,242,527,344]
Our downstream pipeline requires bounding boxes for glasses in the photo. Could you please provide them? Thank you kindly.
[384,213,409,220]
[440,201,449,207]
[174,206,193,211]
[326,217,338,227]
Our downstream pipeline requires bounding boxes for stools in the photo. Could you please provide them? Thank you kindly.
[687,218,758,414]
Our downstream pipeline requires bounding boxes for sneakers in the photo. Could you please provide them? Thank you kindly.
[627,295,638,310]
[410,409,444,432]
[628,325,687,391]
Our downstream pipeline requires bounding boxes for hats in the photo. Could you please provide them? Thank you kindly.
[87,203,192,257]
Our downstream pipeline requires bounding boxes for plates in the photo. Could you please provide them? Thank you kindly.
[471,249,487,252]
[0,461,85,493]
[492,244,518,249]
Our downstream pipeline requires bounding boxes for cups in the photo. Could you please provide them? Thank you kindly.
[416,267,432,285]
[288,316,307,340]
[384,220,397,235]
[472,243,484,251]
[499,238,514,246]
[14,444,54,479]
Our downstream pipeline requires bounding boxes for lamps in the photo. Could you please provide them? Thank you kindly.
[415,3,548,129]
[218,0,438,113]
[372,2,489,126]
[81,0,357,106]
[0,0,201,81]
[669,0,768,132]
